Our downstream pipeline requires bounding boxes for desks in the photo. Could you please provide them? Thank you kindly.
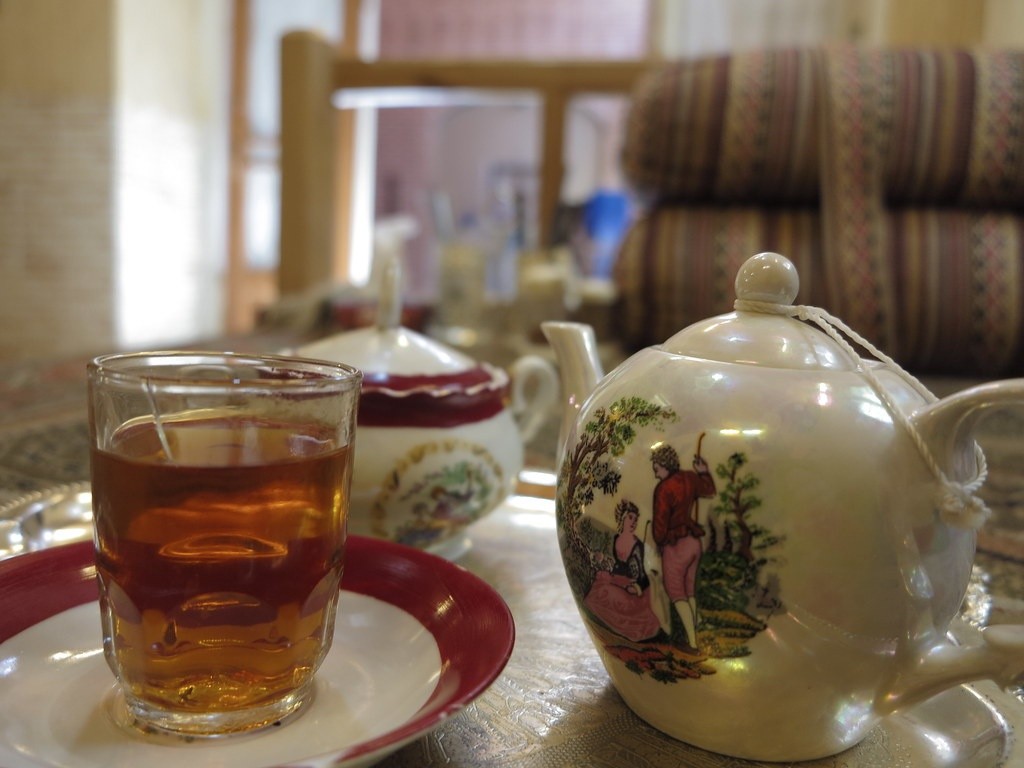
[0,421,1024,768]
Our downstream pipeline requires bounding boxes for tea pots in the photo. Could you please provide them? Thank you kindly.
[541,251,1024,766]
[263,255,559,566]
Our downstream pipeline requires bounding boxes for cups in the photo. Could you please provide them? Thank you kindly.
[85,348,363,747]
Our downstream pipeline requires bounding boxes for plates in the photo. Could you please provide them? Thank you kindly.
[0,534,516,768]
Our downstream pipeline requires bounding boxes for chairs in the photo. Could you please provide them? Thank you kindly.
[609,40,1024,396]
